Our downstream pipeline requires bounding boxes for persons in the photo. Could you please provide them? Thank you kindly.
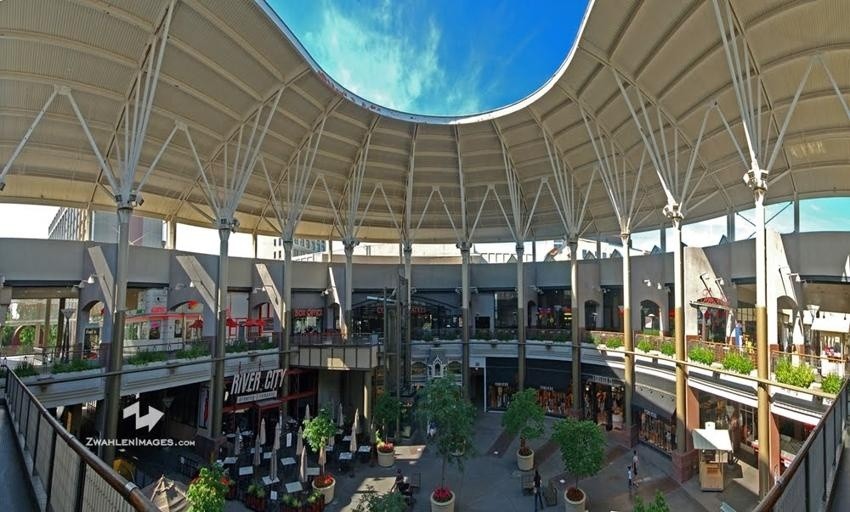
[533,469,544,512]
[395,468,404,490]
[627,465,632,486]
[632,450,639,475]
[429,420,438,440]
[399,483,416,504]
[422,317,433,330]
[426,419,432,439]
[728,319,743,356]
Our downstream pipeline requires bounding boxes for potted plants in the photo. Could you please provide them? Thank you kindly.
[223,479,326,512]
[500,386,612,511]
[297,371,478,512]
